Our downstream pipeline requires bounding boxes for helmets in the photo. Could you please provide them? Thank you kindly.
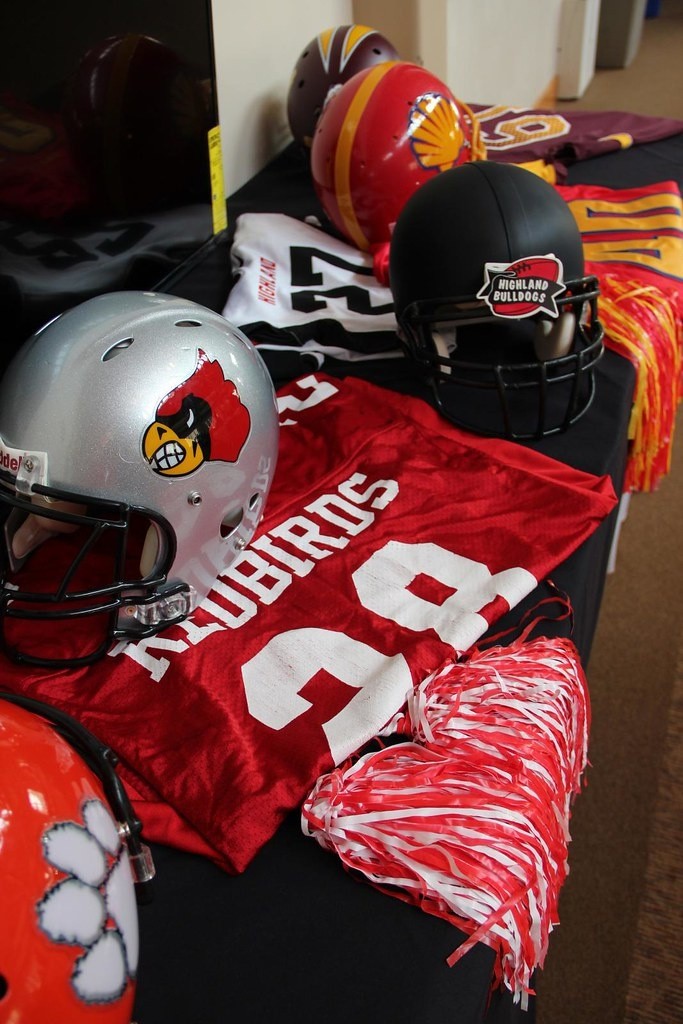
[1,290,279,665]
[288,25,404,165]
[64,30,210,210]
[312,61,488,256]
[1,685,157,1024]
[389,161,605,438]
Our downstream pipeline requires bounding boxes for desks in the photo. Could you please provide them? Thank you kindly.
[127,100,683,1024]
